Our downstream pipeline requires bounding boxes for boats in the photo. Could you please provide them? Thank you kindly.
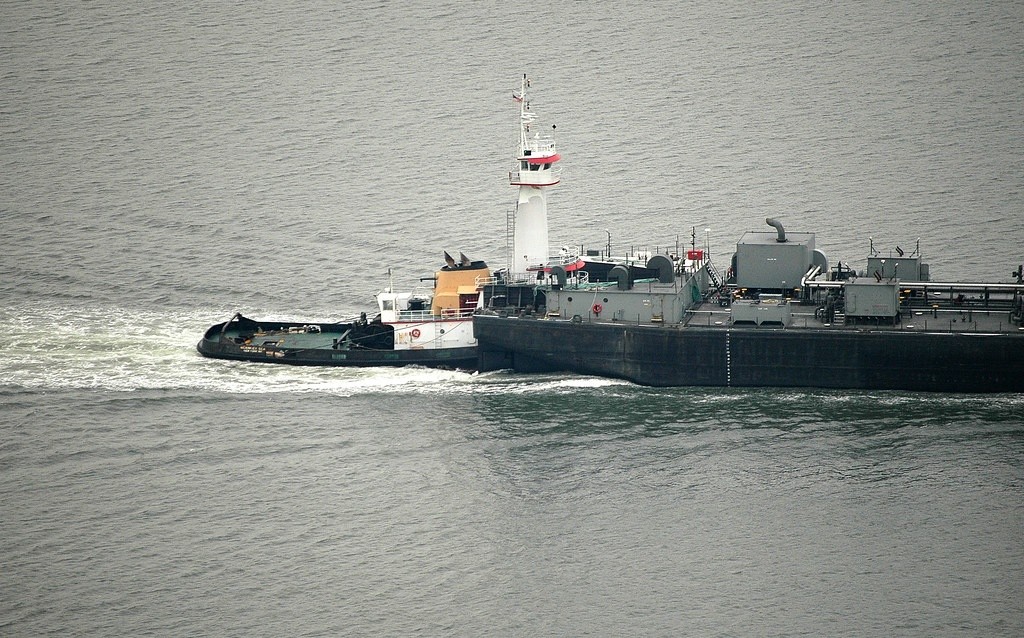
[472,72,1024,395]
[196,250,490,370]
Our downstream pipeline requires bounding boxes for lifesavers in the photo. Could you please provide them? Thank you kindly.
[591,302,604,314]
[411,328,422,339]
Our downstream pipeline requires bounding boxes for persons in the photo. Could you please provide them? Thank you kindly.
[824,292,835,323]
[359,312,367,325]
